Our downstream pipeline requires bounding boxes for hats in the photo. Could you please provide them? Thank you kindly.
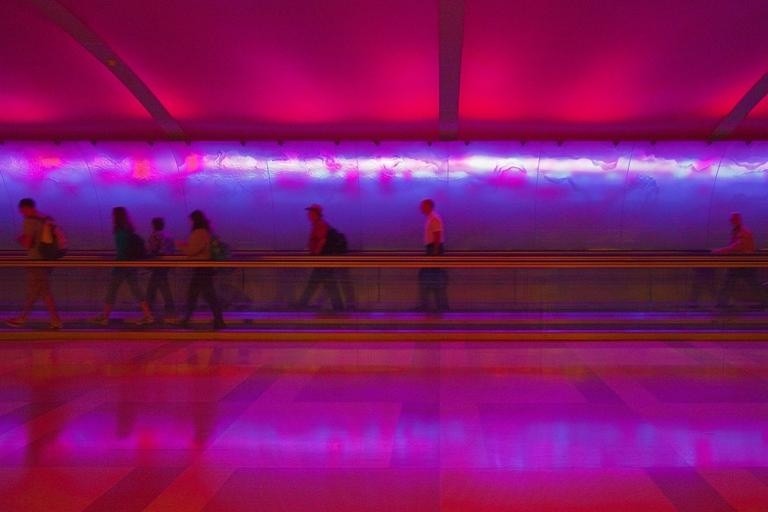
[305,203,322,211]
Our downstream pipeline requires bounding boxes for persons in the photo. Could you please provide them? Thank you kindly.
[294,206,351,313]
[413,197,449,311]
[102,207,229,329]
[7,199,62,329]
[710,211,768,311]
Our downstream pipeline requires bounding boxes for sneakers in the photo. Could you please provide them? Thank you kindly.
[7,319,27,328]
[100,315,226,329]
[48,321,64,329]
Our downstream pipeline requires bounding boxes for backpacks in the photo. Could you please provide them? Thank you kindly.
[210,235,228,262]
[122,229,139,261]
[27,216,67,259]
[327,227,344,255]
[153,234,174,256]
